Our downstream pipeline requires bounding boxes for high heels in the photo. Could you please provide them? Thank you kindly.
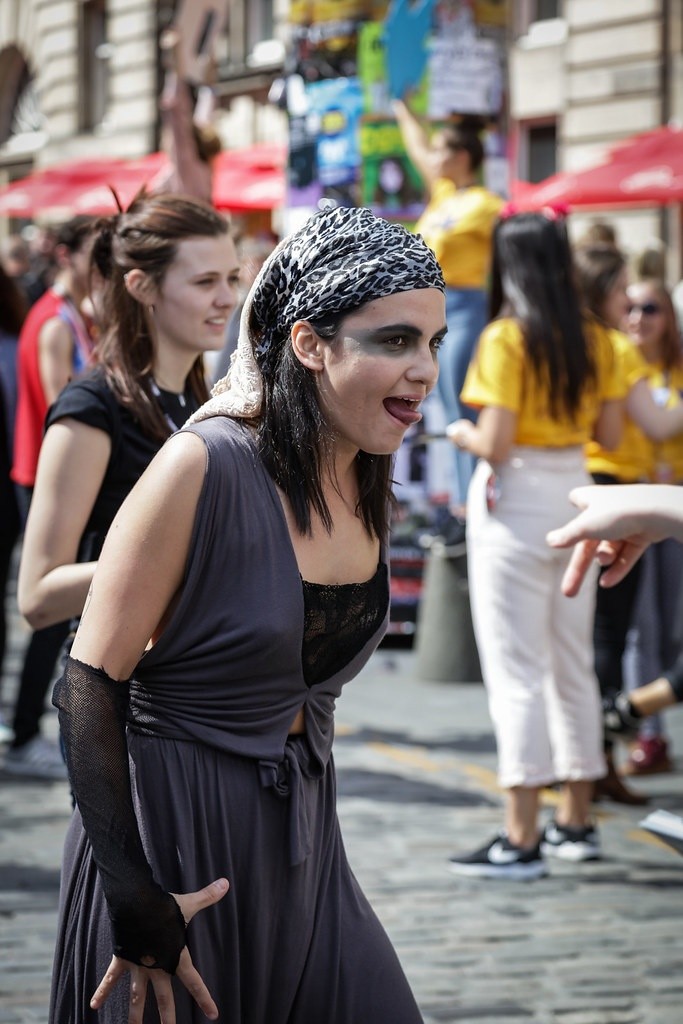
[591,759,649,805]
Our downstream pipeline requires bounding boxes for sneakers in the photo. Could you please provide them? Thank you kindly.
[537,820,602,862]
[600,692,643,745]
[446,829,546,879]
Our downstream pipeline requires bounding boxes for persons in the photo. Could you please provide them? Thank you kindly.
[391,101,683,878]
[0,32,277,782]
[46,207,448,1024]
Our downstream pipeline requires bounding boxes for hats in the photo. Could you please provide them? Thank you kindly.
[574,223,619,255]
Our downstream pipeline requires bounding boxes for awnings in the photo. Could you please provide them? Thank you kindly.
[509,122,683,209]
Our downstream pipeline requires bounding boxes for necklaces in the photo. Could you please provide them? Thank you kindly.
[148,376,186,432]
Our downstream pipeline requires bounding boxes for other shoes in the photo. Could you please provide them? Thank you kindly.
[413,508,455,550]
[7,722,42,761]
[433,519,469,557]
[620,737,673,777]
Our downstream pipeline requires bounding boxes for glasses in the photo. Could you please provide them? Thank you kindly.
[625,299,668,317]
